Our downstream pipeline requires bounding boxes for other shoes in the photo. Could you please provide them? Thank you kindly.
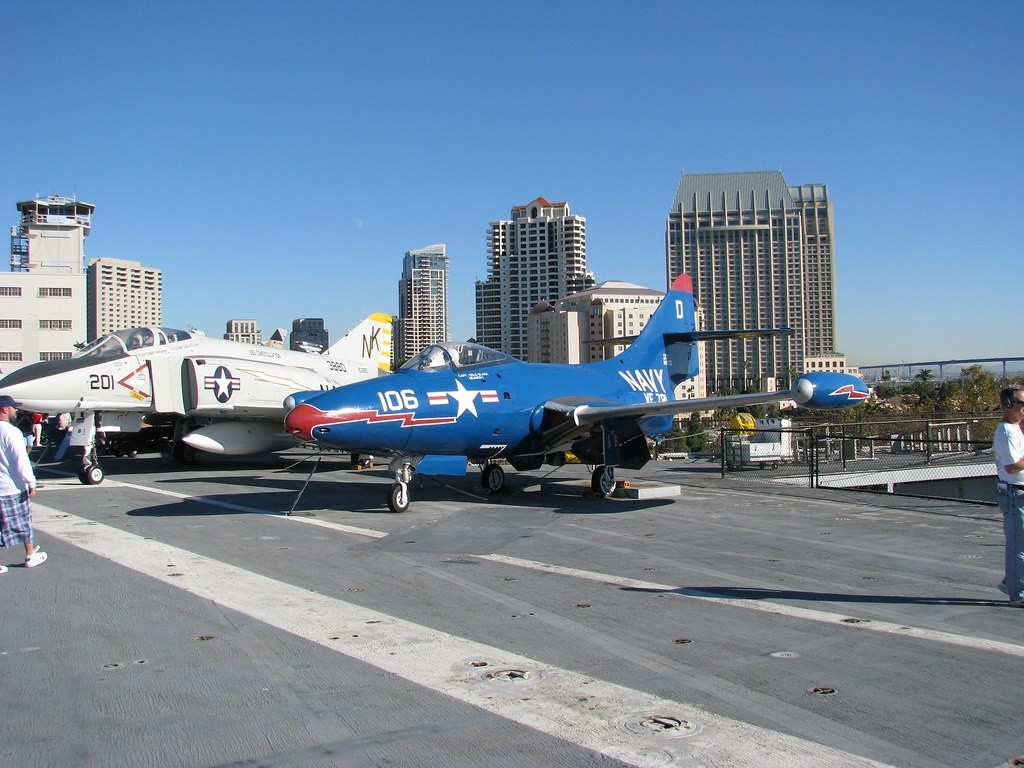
[36,444,42,446]
[997,581,1010,594]
[1009,597,1024,608]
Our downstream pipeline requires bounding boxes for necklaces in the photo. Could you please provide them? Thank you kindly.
[1003,418,1024,438]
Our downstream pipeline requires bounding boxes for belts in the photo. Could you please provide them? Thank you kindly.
[1007,484,1024,490]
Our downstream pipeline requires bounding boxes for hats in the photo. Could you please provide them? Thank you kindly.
[0,396,22,409]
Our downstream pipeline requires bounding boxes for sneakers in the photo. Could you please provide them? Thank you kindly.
[0,565,9,573]
[24,545,48,568]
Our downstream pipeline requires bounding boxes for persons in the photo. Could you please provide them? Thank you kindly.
[0,395,72,574]
[992,386,1024,608]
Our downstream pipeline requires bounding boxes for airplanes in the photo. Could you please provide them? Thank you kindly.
[282,273,870,513]
[0,313,397,485]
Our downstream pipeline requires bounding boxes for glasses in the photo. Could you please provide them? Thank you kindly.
[1011,400,1024,407]
[9,405,19,412]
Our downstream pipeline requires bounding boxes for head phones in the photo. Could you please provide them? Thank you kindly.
[1005,389,1013,410]
